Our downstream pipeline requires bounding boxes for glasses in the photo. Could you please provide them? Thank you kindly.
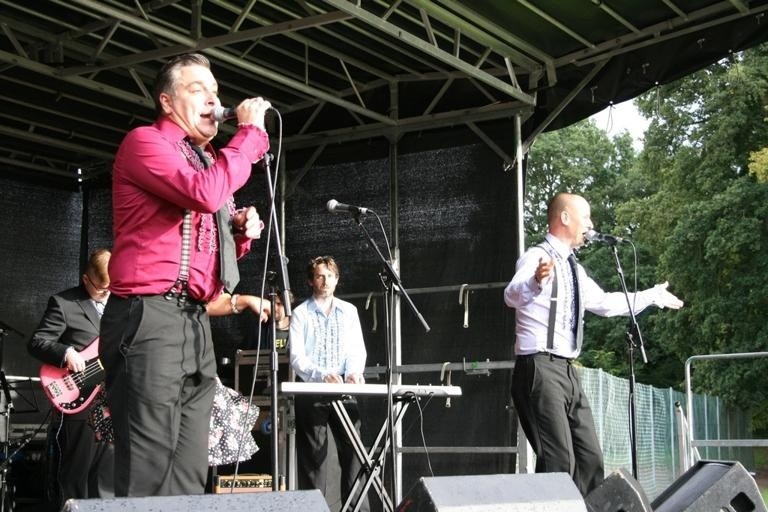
[88,278,108,292]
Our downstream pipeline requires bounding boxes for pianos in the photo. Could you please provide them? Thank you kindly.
[281,382,462,397]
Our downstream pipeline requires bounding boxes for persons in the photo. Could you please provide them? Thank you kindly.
[504,193,685,499]
[289,254,370,512]
[246,292,303,382]
[88,292,273,467]
[99,53,272,496]
[25,248,112,499]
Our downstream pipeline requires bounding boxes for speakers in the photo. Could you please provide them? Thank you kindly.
[651,460,768,512]
[584,468,654,512]
[62,489,330,512]
[397,471,589,512]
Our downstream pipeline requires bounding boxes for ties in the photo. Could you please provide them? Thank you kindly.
[189,142,240,295]
[567,254,583,353]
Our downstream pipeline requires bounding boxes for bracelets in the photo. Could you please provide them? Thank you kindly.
[230,294,241,315]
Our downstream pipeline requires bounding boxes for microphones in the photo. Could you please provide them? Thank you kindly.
[210,105,273,121]
[586,231,624,242]
[327,198,367,214]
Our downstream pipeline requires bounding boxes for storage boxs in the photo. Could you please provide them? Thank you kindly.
[234,349,292,402]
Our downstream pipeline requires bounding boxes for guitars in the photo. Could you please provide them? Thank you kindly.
[38,339,104,415]
